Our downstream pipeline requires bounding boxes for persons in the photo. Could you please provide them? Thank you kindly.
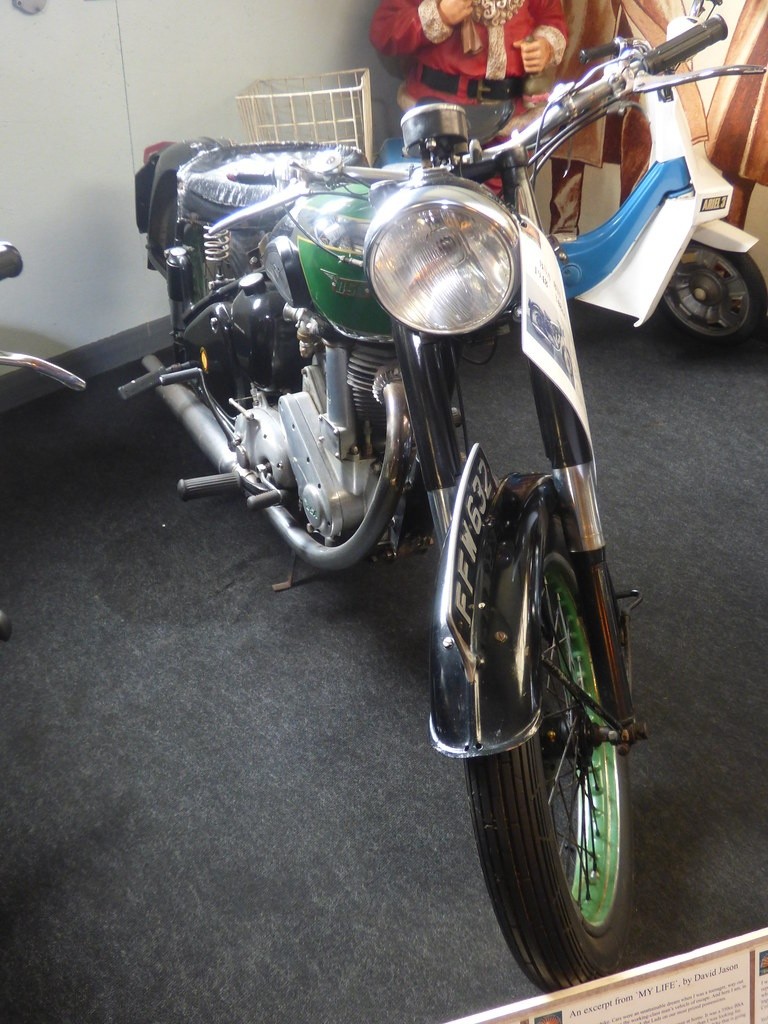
[370,0,570,206]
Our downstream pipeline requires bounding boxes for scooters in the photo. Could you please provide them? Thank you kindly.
[372,0,768,354]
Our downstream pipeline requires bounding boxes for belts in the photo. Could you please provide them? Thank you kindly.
[408,63,527,102]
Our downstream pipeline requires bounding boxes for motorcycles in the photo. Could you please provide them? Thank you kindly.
[116,9,768,998]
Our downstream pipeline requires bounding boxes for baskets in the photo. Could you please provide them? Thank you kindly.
[234,67,375,171]
